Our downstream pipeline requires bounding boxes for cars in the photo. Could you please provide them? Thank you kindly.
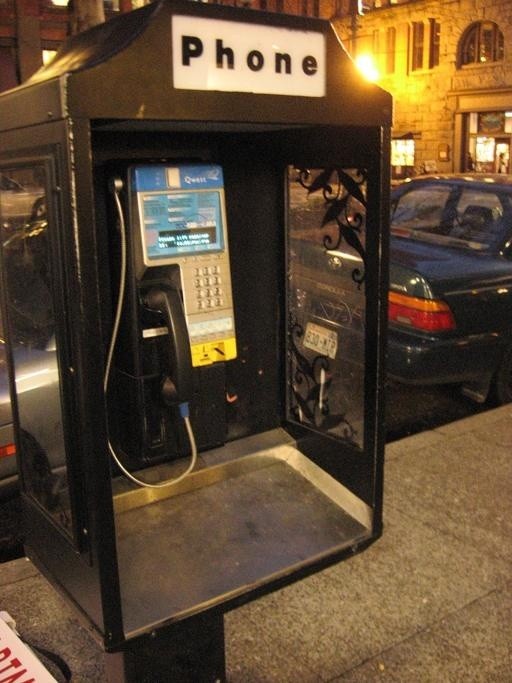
[289,171,511,409]
[0,172,49,226]
[1,328,69,563]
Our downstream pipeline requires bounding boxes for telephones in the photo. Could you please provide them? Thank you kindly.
[122,166,238,391]
